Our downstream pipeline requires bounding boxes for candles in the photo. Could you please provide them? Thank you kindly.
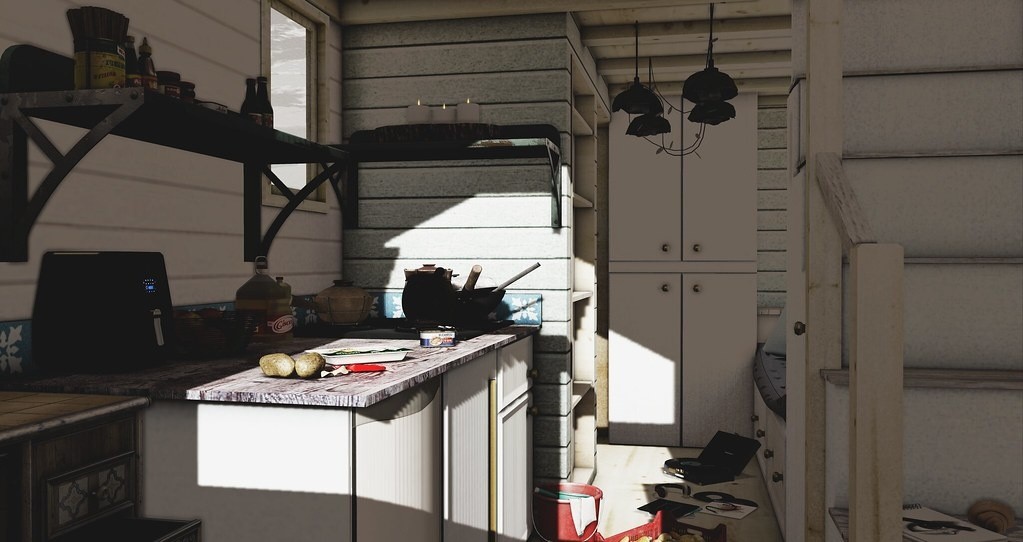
[408,99,431,124]
[431,102,456,123]
[455,98,480,123]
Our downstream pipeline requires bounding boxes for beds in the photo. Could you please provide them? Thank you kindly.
[752,343,786,542]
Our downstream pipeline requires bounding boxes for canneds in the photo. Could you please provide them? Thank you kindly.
[420,330,455,347]
[154,71,195,102]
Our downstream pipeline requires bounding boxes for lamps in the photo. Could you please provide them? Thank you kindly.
[687,42,737,126]
[625,57,673,137]
[680,3,739,105]
[612,20,664,118]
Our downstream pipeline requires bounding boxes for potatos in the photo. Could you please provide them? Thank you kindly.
[259,351,325,378]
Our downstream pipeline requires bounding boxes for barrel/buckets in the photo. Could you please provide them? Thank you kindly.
[533,483,602,542]
[73,38,125,89]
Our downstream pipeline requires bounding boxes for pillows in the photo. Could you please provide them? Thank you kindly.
[762,303,787,356]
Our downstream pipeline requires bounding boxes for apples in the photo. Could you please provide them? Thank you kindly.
[177,307,221,331]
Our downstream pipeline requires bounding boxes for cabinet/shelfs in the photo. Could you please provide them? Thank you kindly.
[0,392,200,542]
[570,104,597,487]
[196,332,534,542]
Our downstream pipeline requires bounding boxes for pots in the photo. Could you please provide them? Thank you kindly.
[312,279,374,324]
[434,265,507,323]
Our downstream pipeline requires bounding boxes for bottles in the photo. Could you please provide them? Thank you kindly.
[137,37,157,90]
[180,81,195,102]
[156,71,180,100]
[239,78,262,125]
[236,256,293,344]
[124,36,141,89]
[257,76,273,129]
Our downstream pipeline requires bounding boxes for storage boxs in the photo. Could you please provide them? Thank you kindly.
[663,430,761,487]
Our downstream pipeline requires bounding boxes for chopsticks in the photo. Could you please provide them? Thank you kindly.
[66,6,129,38]
